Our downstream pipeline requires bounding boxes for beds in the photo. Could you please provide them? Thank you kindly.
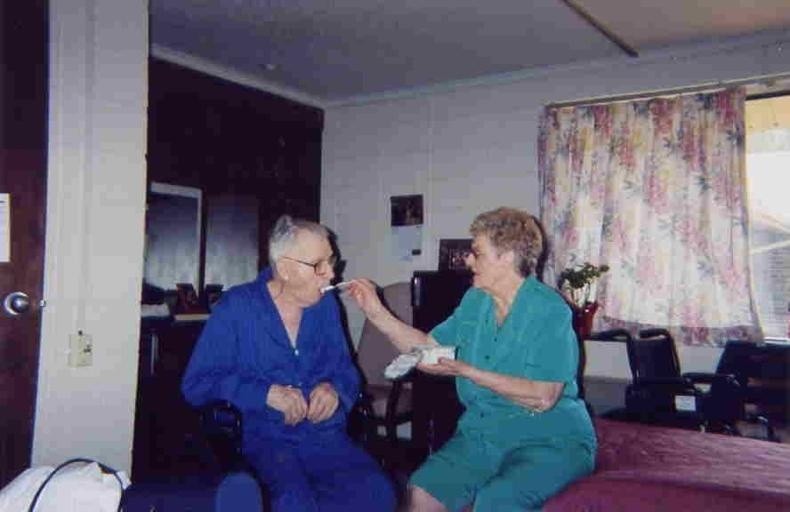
[464,418,790,512]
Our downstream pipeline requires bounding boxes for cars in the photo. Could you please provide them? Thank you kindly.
[750,208,790,315]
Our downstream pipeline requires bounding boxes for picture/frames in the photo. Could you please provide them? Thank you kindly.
[439,239,472,271]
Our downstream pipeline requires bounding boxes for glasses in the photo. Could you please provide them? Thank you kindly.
[278,254,337,276]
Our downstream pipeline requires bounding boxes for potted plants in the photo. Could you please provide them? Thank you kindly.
[556,262,609,337]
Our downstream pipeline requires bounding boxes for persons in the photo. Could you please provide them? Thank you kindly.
[345,204,600,511]
[174,211,400,510]
